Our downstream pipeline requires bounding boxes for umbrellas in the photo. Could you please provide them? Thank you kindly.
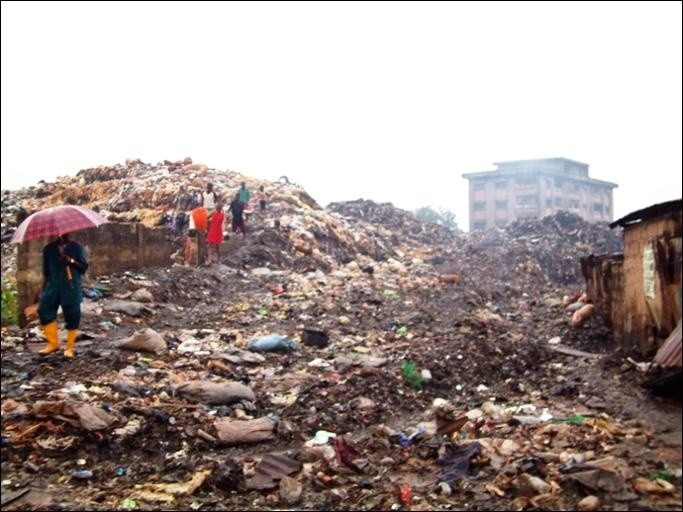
[8,204,110,292]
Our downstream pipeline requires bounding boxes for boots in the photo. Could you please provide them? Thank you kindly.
[63,329,77,356]
[38,321,60,355]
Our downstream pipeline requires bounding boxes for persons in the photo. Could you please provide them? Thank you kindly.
[182,181,249,268]
[35,233,89,358]
[252,185,264,210]
